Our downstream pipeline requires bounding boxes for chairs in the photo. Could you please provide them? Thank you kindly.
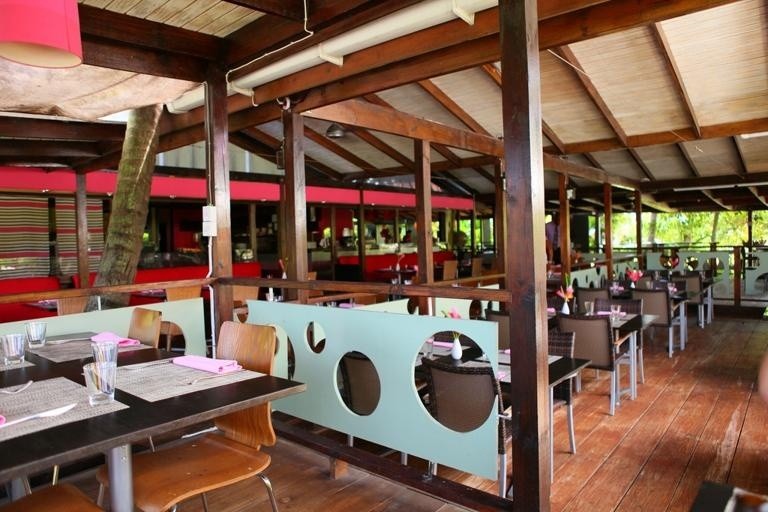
[312,267,722,500]
[91,320,280,511]
[2,480,103,511]
[49,307,163,487]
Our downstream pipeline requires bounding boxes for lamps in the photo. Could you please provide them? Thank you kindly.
[0,0,84,70]
[327,123,346,140]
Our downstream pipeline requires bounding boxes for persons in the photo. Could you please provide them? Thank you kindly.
[380,228,395,243]
[403,230,412,242]
[546,212,560,265]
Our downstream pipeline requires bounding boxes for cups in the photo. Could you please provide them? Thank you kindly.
[93,343,118,363]
[24,321,48,350]
[610,304,622,323]
[1,334,26,366]
[423,341,433,360]
[83,363,116,406]
[583,301,596,315]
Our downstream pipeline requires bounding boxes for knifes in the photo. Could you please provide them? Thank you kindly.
[46,338,91,344]
[123,359,171,369]
[0,402,80,429]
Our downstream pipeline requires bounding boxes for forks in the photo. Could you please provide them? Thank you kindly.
[178,369,246,385]
[0,379,33,394]
